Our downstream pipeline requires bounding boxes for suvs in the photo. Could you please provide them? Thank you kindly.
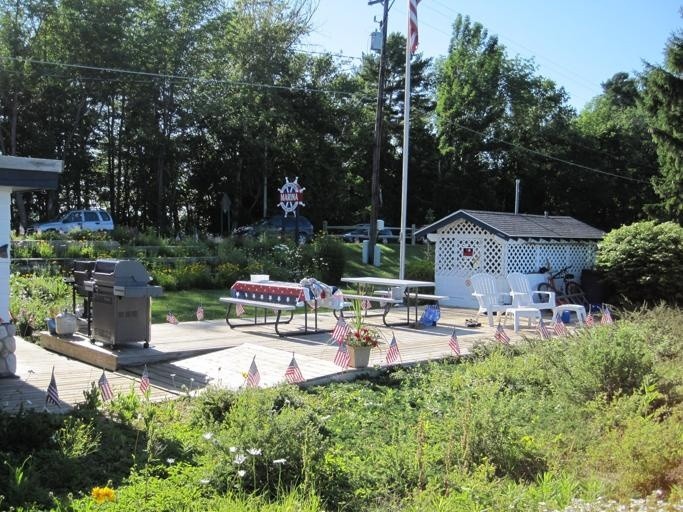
[25,209,114,240]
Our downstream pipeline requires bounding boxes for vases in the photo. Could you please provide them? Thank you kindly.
[18,325,32,337]
[346,344,371,367]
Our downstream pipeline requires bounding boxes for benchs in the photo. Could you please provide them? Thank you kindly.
[219,297,295,337]
[333,294,403,326]
[320,301,353,327]
[373,291,449,325]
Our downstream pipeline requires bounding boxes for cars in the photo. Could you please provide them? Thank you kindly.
[230,214,313,247]
[335,223,393,244]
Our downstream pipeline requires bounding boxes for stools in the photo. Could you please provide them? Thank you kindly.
[550,304,588,328]
[503,307,542,332]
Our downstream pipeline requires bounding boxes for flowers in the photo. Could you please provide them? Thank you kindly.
[337,284,387,351]
[15,307,38,330]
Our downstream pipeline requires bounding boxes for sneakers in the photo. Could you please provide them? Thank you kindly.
[465,319,481,328]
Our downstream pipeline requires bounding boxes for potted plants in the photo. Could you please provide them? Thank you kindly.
[44,302,58,335]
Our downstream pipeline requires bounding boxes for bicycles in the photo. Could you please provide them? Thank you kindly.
[537,256,589,312]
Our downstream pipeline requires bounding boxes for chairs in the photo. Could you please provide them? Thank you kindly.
[507,272,556,327]
[469,273,517,327]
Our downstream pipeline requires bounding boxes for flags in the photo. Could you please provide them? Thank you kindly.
[195,302,204,320]
[235,304,244,317]
[166,311,177,324]
[553,313,564,337]
[361,298,372,310]
[493,322,511,345]
[98,373,114,403]
[586,310,593,326]
[140,367,149,400]
[449,329,460,355]
[333,342,350,370]
[247,361,261,388]
[600,307,613,324]
[386,337,400,365]
[45,372,62,409]
[332,315,347,346]
[285,357,304,384]
[536,317,550,339]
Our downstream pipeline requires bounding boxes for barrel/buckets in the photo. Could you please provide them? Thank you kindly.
[391,286,403,300]
[250,274,270,282]
[377,220,384,231]
[44,318,56,333]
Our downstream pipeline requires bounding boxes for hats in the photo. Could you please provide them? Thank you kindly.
[540,267,549,273]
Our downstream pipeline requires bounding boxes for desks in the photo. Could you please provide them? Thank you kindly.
[229,280,337,336]
[341,277,436,326]
